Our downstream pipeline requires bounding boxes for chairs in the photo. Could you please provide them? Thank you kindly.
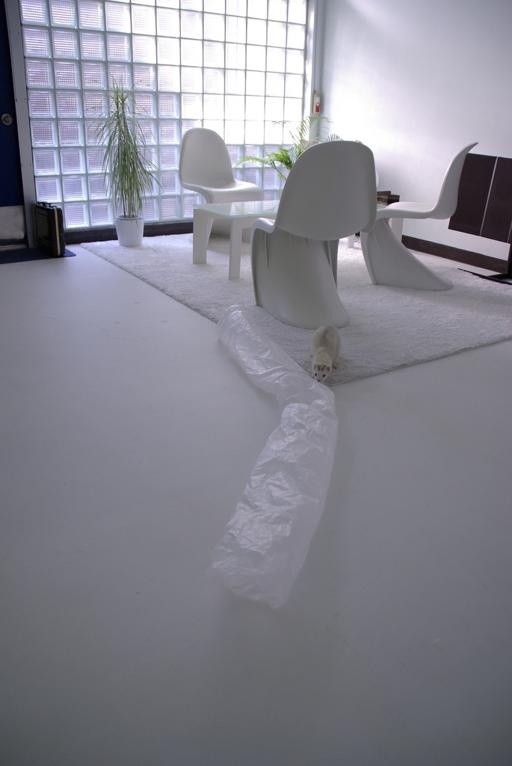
[179,128,263,256]
[250,141,378,329]
[360,142,480,291]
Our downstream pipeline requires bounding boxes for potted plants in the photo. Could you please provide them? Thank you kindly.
[85,77,161,247]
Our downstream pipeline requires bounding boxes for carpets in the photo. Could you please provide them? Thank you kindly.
[0,248,77,264]
[80,233,512,388]
